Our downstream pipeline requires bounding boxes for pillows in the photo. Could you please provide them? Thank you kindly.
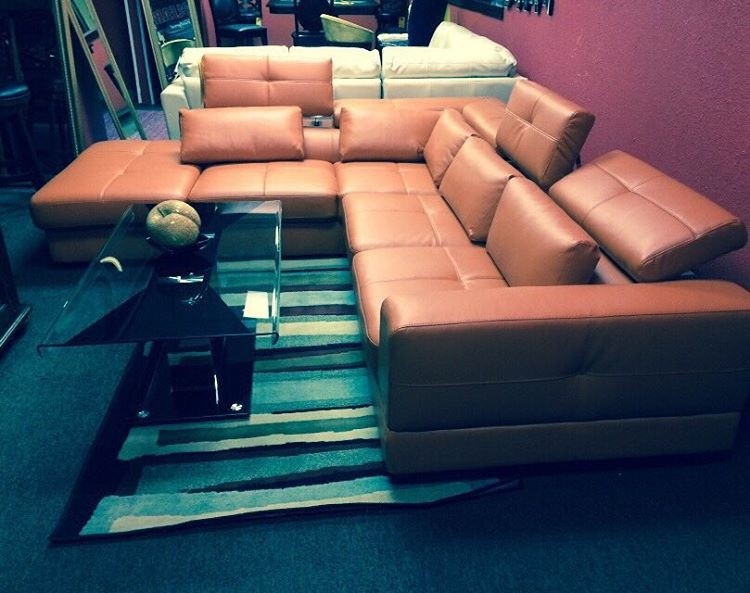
[337,104,445,163]
[421,107,485,188]
[485,175,604,288]
[438,136,528,244]
[177,104,305,164]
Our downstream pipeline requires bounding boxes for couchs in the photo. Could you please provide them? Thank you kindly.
[24,21,750,479]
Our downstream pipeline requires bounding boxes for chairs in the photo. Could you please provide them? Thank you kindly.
[376,0,410,33]
[208,0,269,46]
[291,0,335,46]
[319,14,376,49]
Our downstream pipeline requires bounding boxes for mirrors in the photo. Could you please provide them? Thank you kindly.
[141,0,204,90]
[67,0,148,139]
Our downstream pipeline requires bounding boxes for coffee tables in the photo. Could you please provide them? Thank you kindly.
[34,198,285,428]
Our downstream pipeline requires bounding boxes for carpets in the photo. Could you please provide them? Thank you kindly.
[45,255,525,548]
